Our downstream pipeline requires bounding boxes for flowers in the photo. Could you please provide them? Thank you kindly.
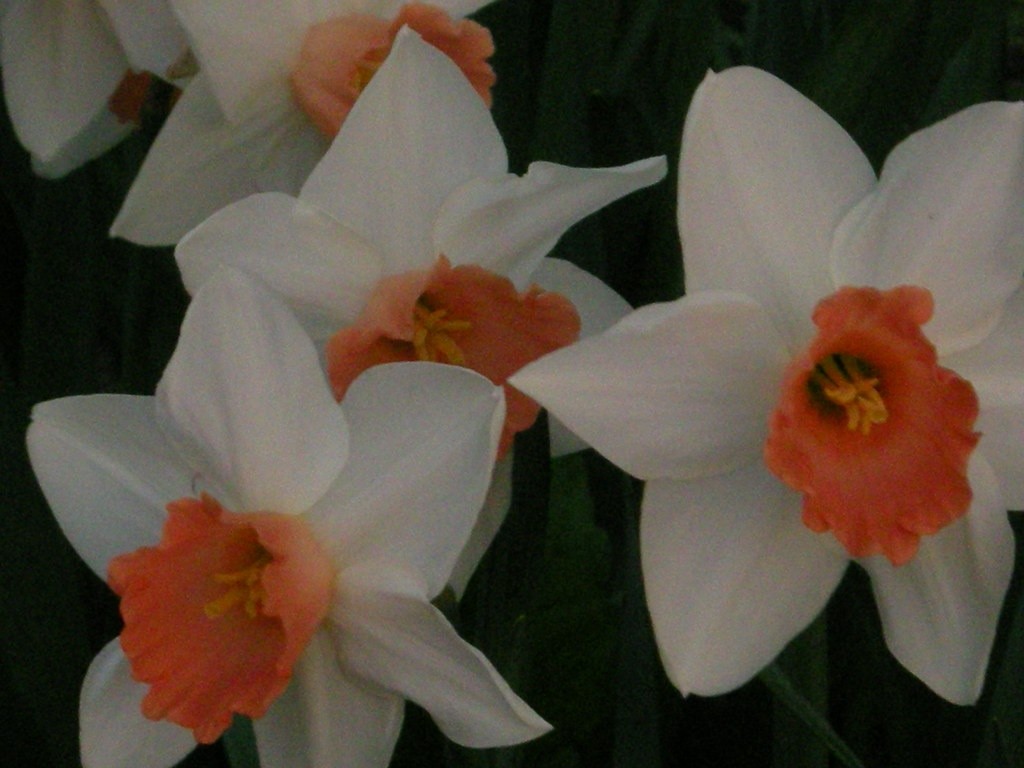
[0,0,1023,768]
